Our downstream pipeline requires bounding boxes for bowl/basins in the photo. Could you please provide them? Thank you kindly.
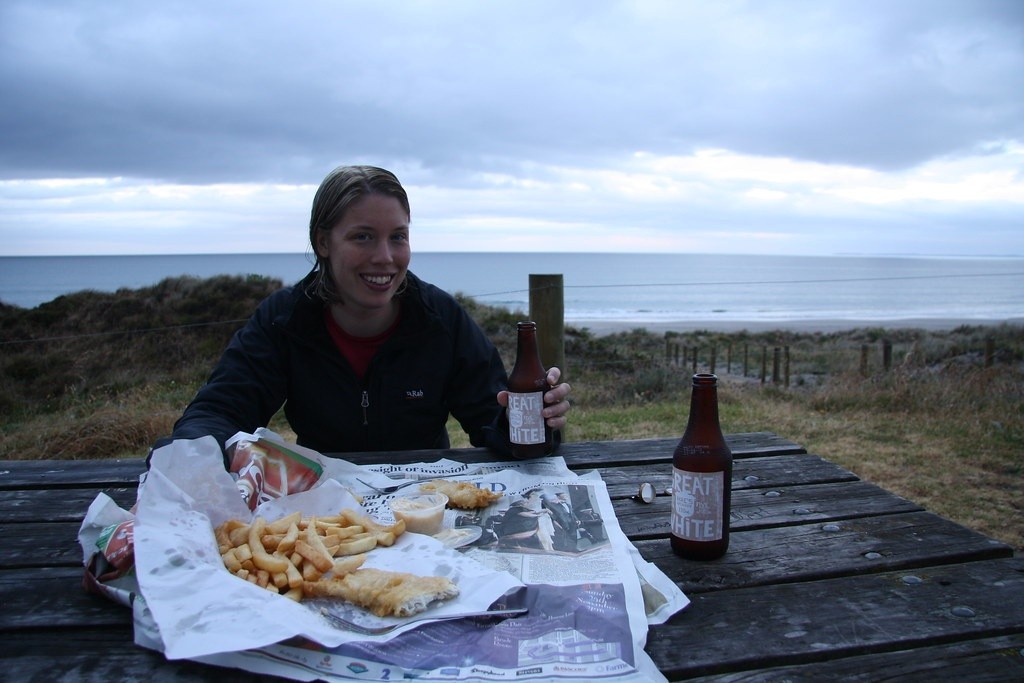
[388,490,449,534]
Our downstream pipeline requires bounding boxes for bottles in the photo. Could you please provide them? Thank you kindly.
[670,373,733,561]
[507,321,554,459]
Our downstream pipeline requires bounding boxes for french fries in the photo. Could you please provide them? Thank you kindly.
[215,508,406,603]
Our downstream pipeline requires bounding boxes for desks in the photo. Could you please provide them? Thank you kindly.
[0,431,1023,683]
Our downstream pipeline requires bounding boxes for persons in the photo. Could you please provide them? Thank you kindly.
[145,165,572,472]
[486,500,554,549]
[547,492,581,552]
